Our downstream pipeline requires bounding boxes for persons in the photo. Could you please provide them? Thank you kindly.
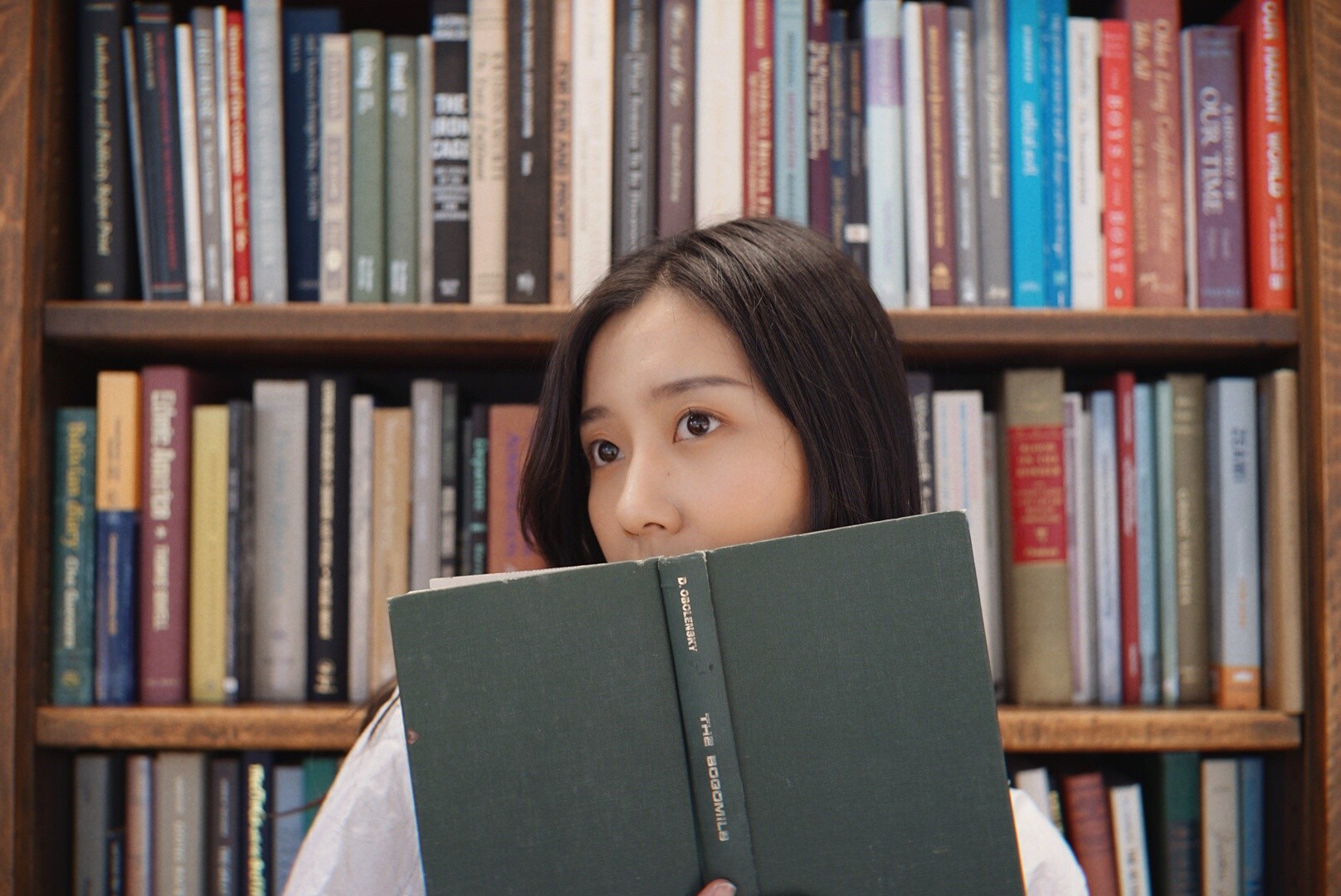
[281,216,1090,896]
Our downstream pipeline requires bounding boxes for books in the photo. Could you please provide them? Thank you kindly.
[386,507,1026,896]
[1016,749,1269,896]
[52,364,1307,714]
[82,0,1305,316]
[70,744,346,896]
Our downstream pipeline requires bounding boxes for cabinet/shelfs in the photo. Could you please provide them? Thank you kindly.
[0,0,1341,895]
